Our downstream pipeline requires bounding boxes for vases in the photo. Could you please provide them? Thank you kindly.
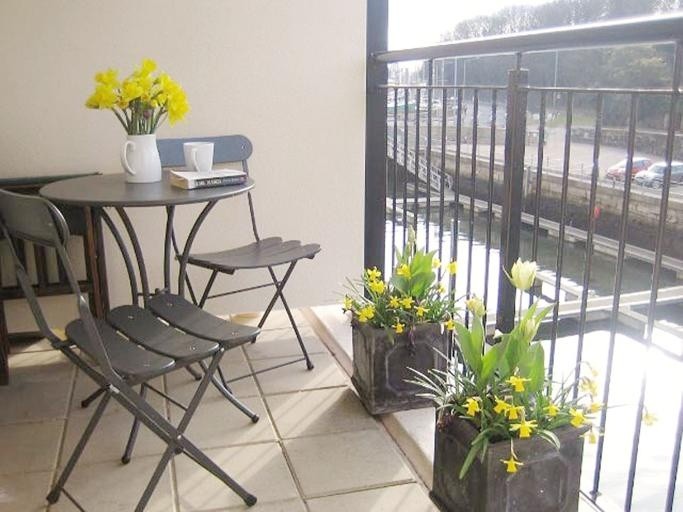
[83,61,195,137]
[339,223,465,337]
[402,255,616,481]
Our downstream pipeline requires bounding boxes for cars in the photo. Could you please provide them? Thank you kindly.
[182,141,215,173]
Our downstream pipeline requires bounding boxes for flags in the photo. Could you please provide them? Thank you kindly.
[338,306,461,419]
[114,132,165,184]
[423,403,594,512]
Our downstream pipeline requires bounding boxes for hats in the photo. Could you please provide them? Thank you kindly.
[386,83,560,143]
[605,156,653,183]
[634,161,682,190]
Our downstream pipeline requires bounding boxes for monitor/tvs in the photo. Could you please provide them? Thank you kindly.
[168,167,247,192]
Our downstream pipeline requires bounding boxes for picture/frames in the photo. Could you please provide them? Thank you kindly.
[38,167,261,466]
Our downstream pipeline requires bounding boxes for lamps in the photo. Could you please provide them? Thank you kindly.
[154,130,331,398]
[0,168,113,391]
[0,186,263,512]
